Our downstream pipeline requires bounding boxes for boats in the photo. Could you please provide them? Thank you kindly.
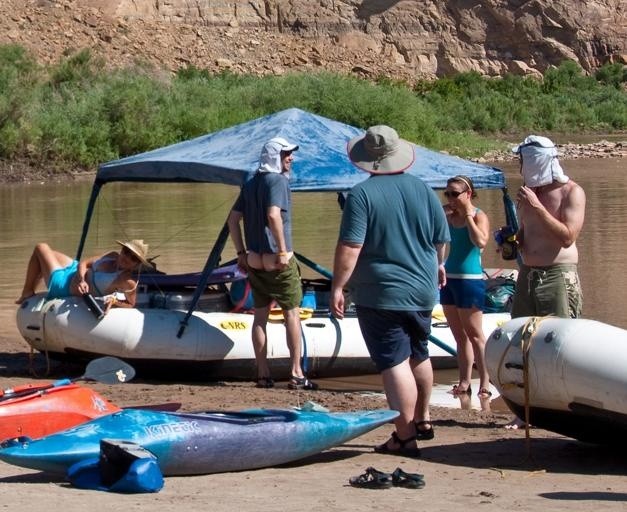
[8,258,527,369]
[481,315,627,447]
[0,396,403,500]
[0,376,125,459]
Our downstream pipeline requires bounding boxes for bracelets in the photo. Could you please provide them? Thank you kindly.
[465,214,473,216]
[277,253,287,257]
[236,250,246,255]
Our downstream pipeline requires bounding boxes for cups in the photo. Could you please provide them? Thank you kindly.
[501,233,516,260]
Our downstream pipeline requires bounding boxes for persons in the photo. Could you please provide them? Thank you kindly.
[503,135,587,319]
[440,176,490,398]
[227,137,317,390]
[331,125,447,454]
[15,240,149,313]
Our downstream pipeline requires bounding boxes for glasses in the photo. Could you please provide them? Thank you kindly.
[121,244,139,264]
[443,187,468,198]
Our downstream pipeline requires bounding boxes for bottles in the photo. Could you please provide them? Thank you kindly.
[82,291,104,321]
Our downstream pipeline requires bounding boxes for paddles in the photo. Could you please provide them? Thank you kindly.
[0,356,136,401]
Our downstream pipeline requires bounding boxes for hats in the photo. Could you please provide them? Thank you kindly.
[114,238,154,270]
[345,123,418,175]
[510,134,555,166]
[264,137,299,152]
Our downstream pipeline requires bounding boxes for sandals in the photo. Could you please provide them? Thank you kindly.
[348,466,394,490]
[386,466,427,490]
[448,385,494,399]
[413,420,435,441]
[374,431,422,458]
[255,376,274,389]
[287,374,319,391]
